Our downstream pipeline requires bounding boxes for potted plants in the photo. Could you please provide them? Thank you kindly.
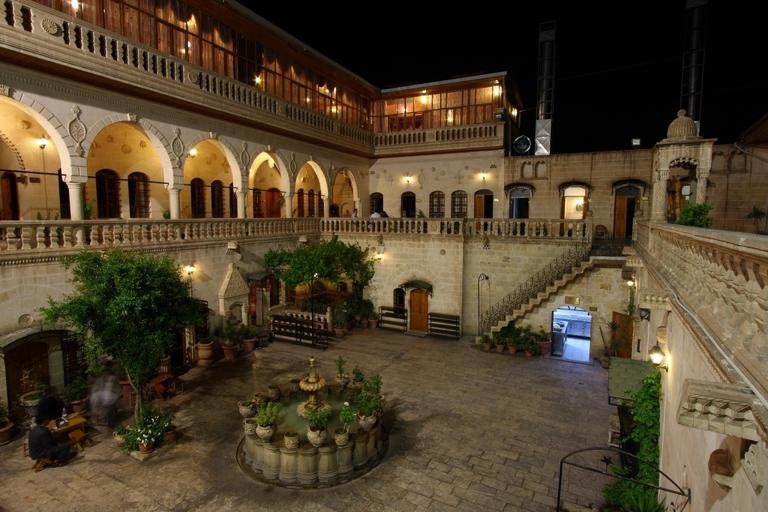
[599,320,620,369]
[113,401,179,454]
[64,375,90,414]
[196,298,386,450]
[0,401,15,446]
[481,324,552,357]
[20,382,54,417]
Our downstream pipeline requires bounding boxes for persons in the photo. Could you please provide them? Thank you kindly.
[351,208,358,218]
[370,208,382,218]
[28,414,65,467]
[377,209,389,218]
[34,386,66,418]
[89,369,122,431]
